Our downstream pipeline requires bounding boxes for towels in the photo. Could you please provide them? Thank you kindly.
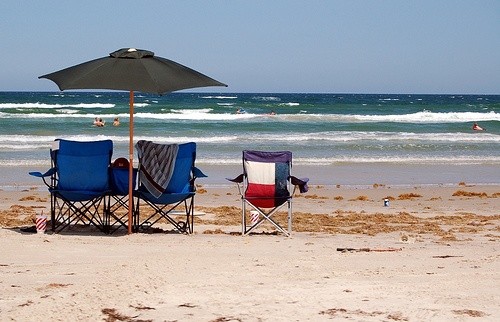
[134,140,180,200]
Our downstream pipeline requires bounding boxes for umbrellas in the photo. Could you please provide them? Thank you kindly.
[37,46,229,234]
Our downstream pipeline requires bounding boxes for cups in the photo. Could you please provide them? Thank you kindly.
[36,214,47,233]
[250,211,259,225]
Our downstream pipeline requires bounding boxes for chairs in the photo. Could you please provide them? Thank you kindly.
[225,151,310,237]
[28,138,113,235]
[132,140,209,234]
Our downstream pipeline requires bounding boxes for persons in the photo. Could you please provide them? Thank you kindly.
[93,116,100,125]
[473,122,485,132]
[95,119,106,128]
[236,107,246,114]
[263,111,276,115]
[111,117,121,127]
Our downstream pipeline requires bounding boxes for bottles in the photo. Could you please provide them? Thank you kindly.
[384,198,389,206]
